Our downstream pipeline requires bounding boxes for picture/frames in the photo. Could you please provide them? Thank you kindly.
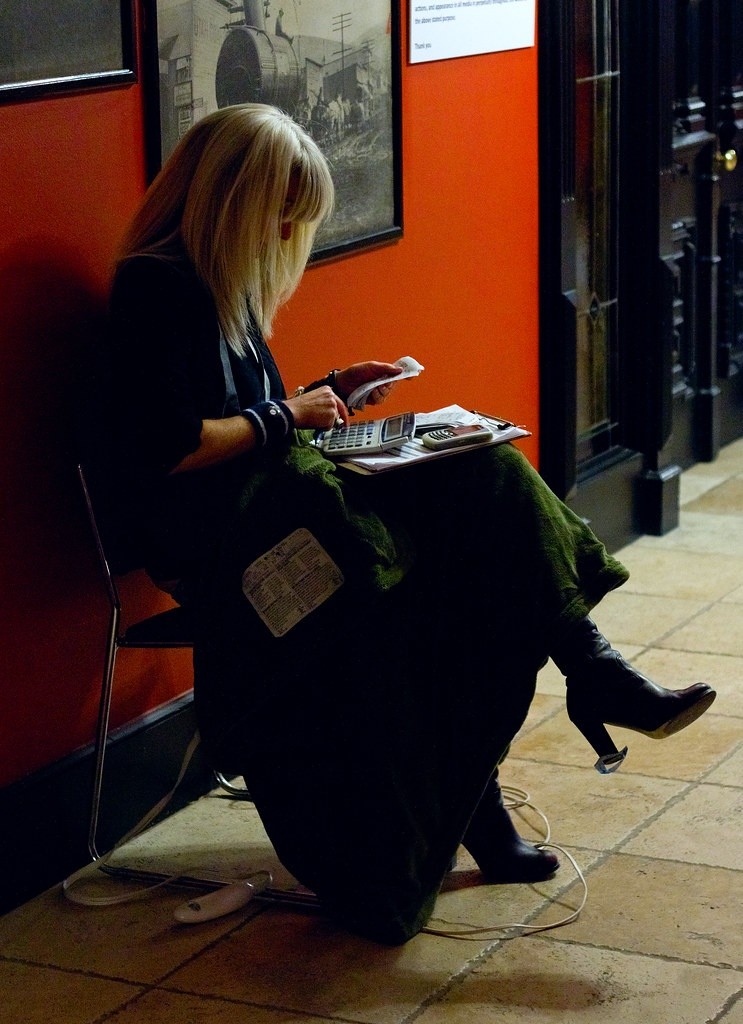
[138,0,403,269]
[0,0,138,103]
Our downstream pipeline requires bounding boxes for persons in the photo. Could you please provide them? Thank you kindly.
[95,102,715,941]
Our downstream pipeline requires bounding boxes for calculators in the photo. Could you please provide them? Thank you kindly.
[322,411,418,460]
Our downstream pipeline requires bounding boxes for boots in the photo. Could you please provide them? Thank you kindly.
[550,616,717,765]
[450,768,560,880]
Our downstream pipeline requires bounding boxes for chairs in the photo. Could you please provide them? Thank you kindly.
[72,463,321,914]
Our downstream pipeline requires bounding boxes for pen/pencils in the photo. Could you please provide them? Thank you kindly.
[327,370,334,394]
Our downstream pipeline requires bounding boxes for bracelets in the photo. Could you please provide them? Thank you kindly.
[238,398,297,452]
[303,367,349,401]
